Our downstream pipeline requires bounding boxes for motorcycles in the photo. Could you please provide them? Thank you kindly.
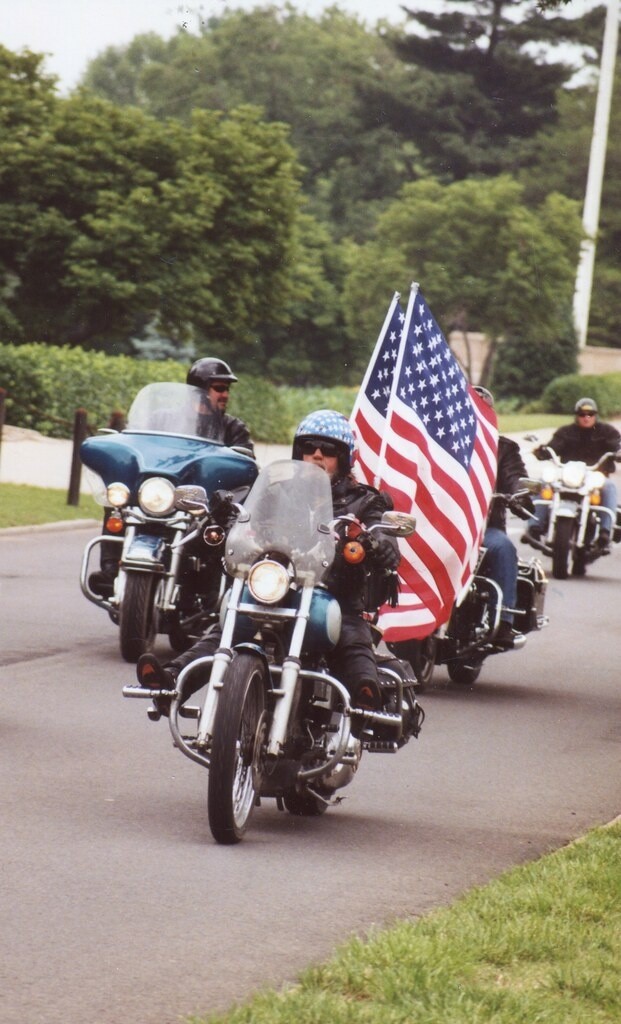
[80,383,256,665]
[396,429,556,679]
[520,435,620,578]
[122,457,426,842]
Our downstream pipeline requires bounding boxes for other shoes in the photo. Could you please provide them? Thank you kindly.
[521,532,541,542]
[351,678,381,738]
[136,653,174,716]
[492,629,515,648]
[598,532,609,546]
[89,571,114,596]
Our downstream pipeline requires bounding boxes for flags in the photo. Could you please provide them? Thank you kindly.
[347,280,498,643]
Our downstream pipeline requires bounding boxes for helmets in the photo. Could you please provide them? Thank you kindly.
[292,409,355,473]
[472,385,494,408]
[575,399,597,413]
[187,357,238,392]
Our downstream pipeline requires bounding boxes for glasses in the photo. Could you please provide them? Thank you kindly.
[209,384,231,393]
[301,440,344,458]
[577,410,596,416]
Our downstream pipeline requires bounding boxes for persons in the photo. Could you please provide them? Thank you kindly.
[521,397,621,549]
[470,386,536,648]
[136,409,402,734]
[89,356,256,614]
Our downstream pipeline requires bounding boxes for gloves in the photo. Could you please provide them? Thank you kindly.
[366,540,400,571]
